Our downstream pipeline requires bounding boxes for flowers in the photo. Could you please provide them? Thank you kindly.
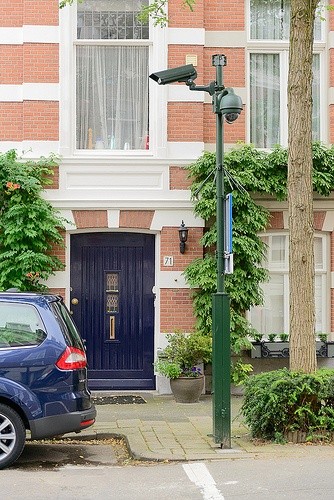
[152,330,213,379]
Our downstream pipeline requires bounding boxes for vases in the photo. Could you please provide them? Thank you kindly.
[170,375,204,403]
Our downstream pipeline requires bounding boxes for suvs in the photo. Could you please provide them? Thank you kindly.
[0,290,97,470]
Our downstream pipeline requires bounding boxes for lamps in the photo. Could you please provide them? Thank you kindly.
[178,220,189,255]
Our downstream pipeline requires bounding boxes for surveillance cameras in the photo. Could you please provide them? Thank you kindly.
[219,93,242,124]
[148,64,196,85]
